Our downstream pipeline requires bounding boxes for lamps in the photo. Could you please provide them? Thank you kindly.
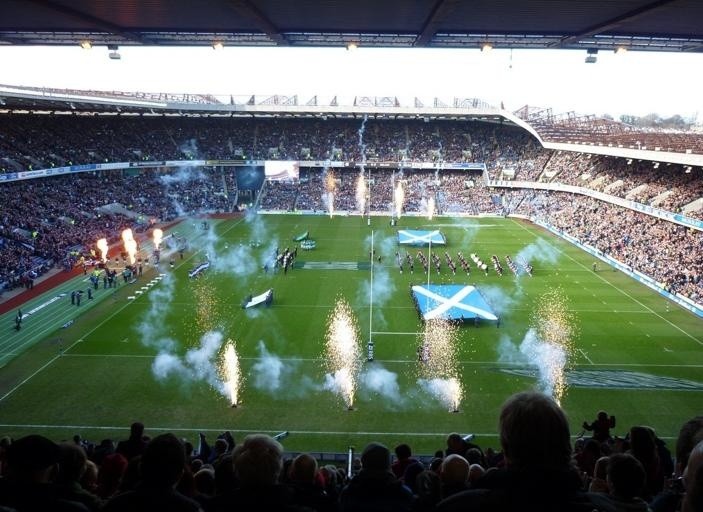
[480,42,492,52]
[585,48,599,63]
[346,40,357,51]
[108,44,120,59]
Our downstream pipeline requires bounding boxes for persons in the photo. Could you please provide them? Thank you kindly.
[0,393,703,512]
[0,113,703,330]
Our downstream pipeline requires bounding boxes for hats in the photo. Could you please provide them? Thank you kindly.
[104,454,128,476]
[6,435,64,474]
